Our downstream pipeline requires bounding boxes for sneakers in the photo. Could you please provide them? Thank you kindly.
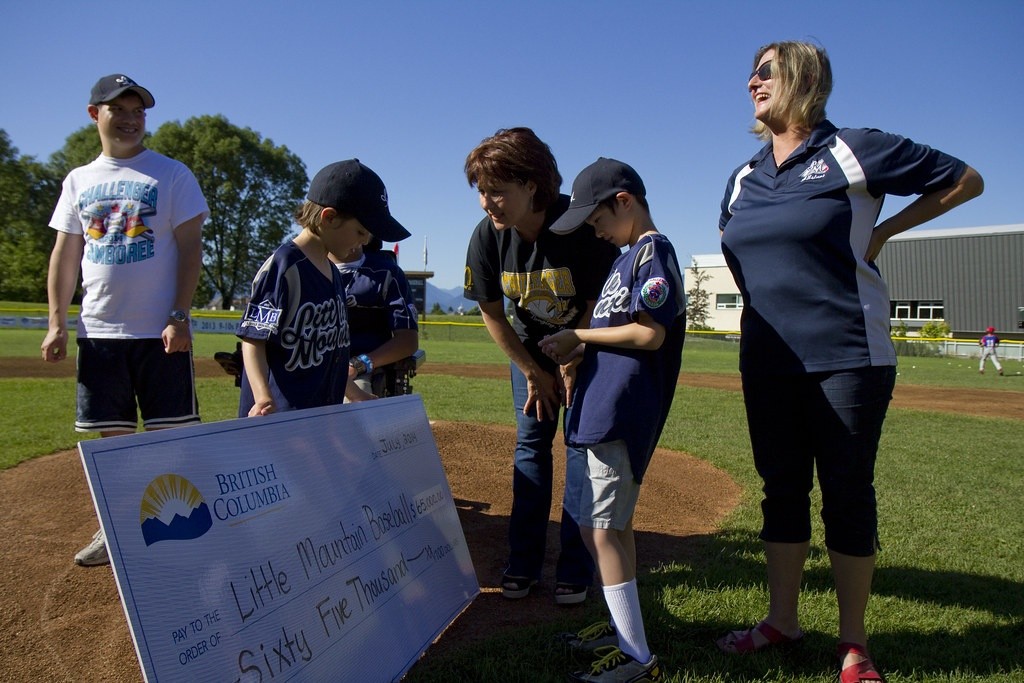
[568,621,618,648]
[567,645,662,683]
[74,529,110,567]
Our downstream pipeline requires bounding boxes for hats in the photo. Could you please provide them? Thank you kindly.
[549,157,646,235]
[307,158,411,242]
[89,74,155,108]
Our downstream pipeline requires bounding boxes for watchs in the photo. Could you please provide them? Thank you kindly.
[169,309,190,325]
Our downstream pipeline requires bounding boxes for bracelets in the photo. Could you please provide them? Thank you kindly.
[349,355,374,376]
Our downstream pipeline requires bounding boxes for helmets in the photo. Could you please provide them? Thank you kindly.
[986,326,997,332]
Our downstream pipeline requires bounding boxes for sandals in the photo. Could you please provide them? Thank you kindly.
[501,575,538,598]
[555,582,588,603]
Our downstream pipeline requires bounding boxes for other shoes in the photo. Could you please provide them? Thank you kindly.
[998,369,1003,376]
[980,370,984,374]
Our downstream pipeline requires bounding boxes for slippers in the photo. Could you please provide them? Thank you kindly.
[835,641,884,683]
[715,620,804,654]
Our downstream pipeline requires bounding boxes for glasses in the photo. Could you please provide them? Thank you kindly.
[749,60,799,81]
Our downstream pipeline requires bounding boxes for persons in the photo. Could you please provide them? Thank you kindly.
[718,40,984,683]
[39,74,211,565]
[538,158,687,683]
[327,230,420,406]
[462,126,622,605]
[979,327,1004,375]
[235,159,412,417]
